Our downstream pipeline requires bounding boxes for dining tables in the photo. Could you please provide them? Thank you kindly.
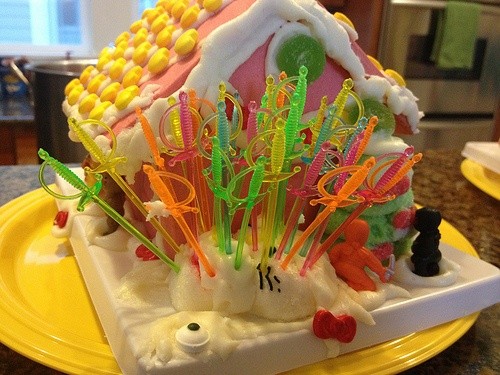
[0,148,500,375]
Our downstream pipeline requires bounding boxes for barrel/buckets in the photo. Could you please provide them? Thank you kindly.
[25,59,100,163]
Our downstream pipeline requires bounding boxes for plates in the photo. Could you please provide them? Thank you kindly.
[460,158,500,200]
[0,186,482,375]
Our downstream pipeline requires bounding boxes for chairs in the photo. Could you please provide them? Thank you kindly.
[0,56,36,165]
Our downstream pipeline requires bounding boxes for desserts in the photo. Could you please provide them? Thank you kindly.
[38,0,459,370]
